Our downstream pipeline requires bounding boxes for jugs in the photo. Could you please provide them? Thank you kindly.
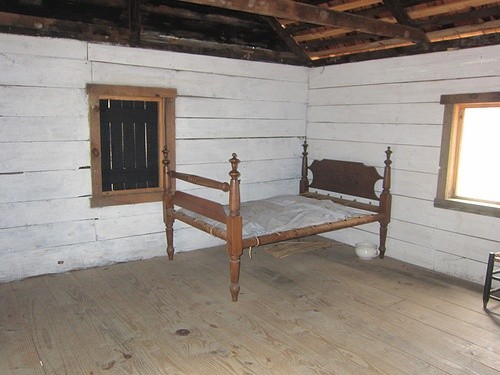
[354,241,380,260]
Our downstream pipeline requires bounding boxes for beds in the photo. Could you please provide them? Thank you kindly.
[162,141,392,301]
